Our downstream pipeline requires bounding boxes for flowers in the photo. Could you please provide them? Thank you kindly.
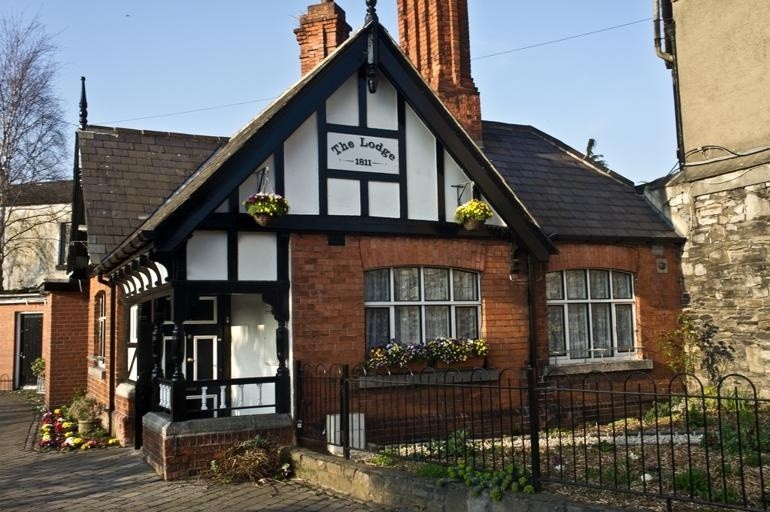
[367,336,493,369]
[453,198,493,225]
[241,191,290,218]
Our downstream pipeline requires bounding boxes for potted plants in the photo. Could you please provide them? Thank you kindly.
[30,357,46,394]
[66,395,106,433]
[254,215,279,228]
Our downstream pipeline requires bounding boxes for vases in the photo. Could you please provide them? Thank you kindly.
[462,216,485,231]
[375,357,428,373]
